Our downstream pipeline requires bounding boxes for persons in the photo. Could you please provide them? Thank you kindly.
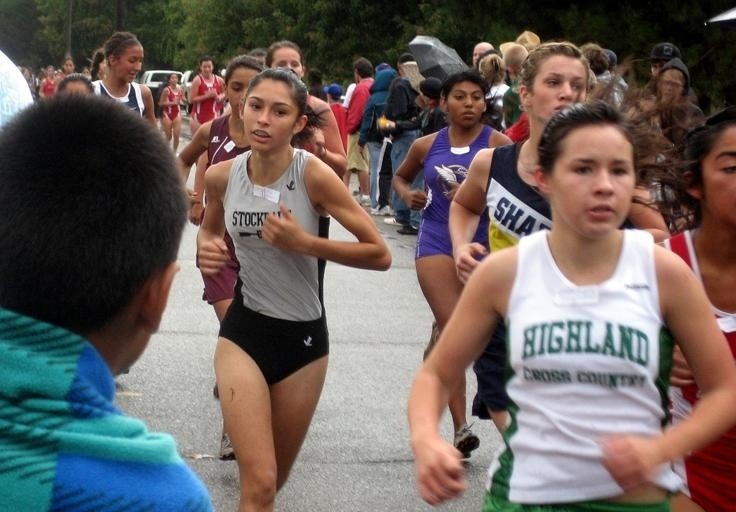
[371,30,736,512]
[0,94,211,512]
[169,41,393,509]
[0,29,192,158]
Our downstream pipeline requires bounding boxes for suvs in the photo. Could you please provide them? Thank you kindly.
[140,71,184,89]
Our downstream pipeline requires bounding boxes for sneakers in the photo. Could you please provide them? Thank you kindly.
[353,194,393,215]
[397,224,418,235]
[384,217,409,225]
[453,424,480,458]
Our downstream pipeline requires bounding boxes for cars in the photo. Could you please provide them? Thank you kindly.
[180,70,199,114]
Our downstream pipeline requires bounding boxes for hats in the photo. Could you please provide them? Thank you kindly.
[645,42,681,61]
